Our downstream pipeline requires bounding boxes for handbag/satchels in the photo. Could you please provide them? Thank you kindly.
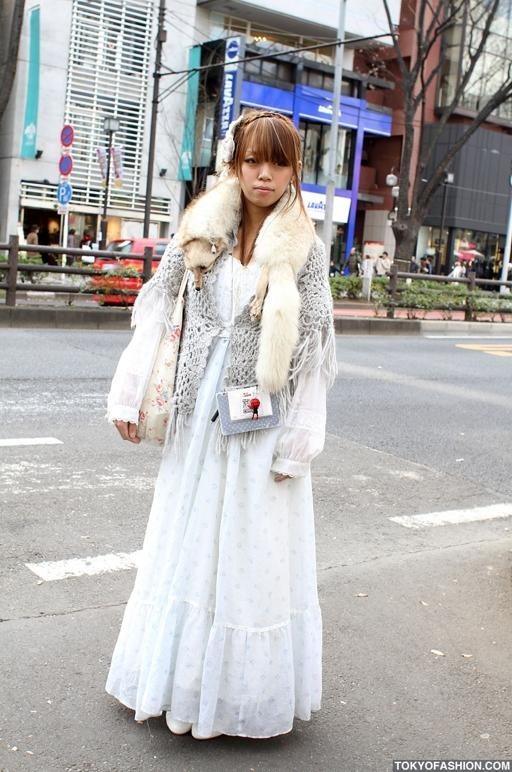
[133,295,185,448]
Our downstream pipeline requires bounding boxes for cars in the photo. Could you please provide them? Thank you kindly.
[92,238,171,306]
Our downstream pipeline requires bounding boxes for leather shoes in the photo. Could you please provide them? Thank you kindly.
[165,710,223,741]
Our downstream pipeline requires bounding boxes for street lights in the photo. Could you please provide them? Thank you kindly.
[434,173,454,275]
[98,118,119,250]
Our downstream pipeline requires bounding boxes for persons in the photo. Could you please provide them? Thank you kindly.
[26,224,40,257]
[66,228,94,267]
[448,261,466,285]
[341,247,392,277]
[410,254,433,274]
[105,108,339,740]
